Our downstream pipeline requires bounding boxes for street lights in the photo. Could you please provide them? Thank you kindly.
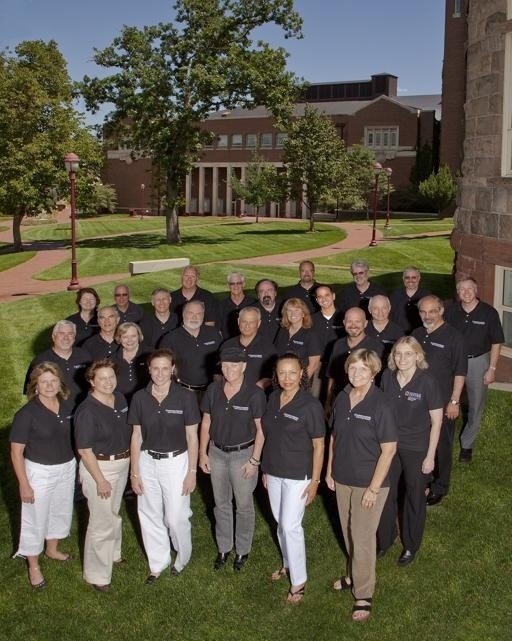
[139,181,146,220]
[383,168,394,227]
[369,161,383,247]
[63,151,82,290]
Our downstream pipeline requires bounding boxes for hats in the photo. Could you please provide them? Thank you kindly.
[215,347,248,366]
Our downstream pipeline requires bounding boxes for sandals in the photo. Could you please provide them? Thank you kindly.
[333,576,352,590]
[271,566,289,581]
[286,582,306,603]
[351,598,372,621]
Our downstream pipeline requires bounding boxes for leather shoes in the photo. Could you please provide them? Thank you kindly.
[171,566,181,575]
[233,553,249,570]
[27,564,47,590]
[425,489,449,506]
[397,547,417,567]
[42,550,74,562]
[88,580,113,591]
[112,557,127,567]
[374,547,387,559]
[213,551,231,571]
[459,447,473,463]
[143,573,161,585]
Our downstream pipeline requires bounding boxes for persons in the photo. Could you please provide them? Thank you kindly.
[110,282,142,322]
[65,286,100,346]
[387,264,439,335]
[307,283,349,353]
[106,324,155,395]
[370,335,444,566]
[139,288,180,347]
[215,305,271,388]
[447,278,504,463]
[365,294,405,352]
[127,347,199,586]
[274,298,321,392]
[252,278,282,343]
[412,290,468,505]
[75,360,130,593]
[327,308,386,414]
[262,350,326,608]
[326,348,399,621]
[283,260,323,315]
[199,343,268,572]
[216,271,258,339]
[80,307,123,358]
[11,363,79,588]
[171,267,217,327]
[339,259,382,321]
[159,303,221,388]
[22,317,91,418]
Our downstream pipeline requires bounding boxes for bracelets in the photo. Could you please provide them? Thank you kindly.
[187,469,197,474]
[130,474,139,480]
[249,457,262,466]
[369,487,381,495]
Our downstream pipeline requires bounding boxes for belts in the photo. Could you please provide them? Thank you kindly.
[212,439,258,453]
[468,349,490,359]
[95,450,132,461]
[177,380,208,392]
[147,448,189,460]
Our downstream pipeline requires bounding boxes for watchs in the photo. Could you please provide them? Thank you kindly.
[448,399,460,406]
[489,365,497,371]
[312,479,321,484]
